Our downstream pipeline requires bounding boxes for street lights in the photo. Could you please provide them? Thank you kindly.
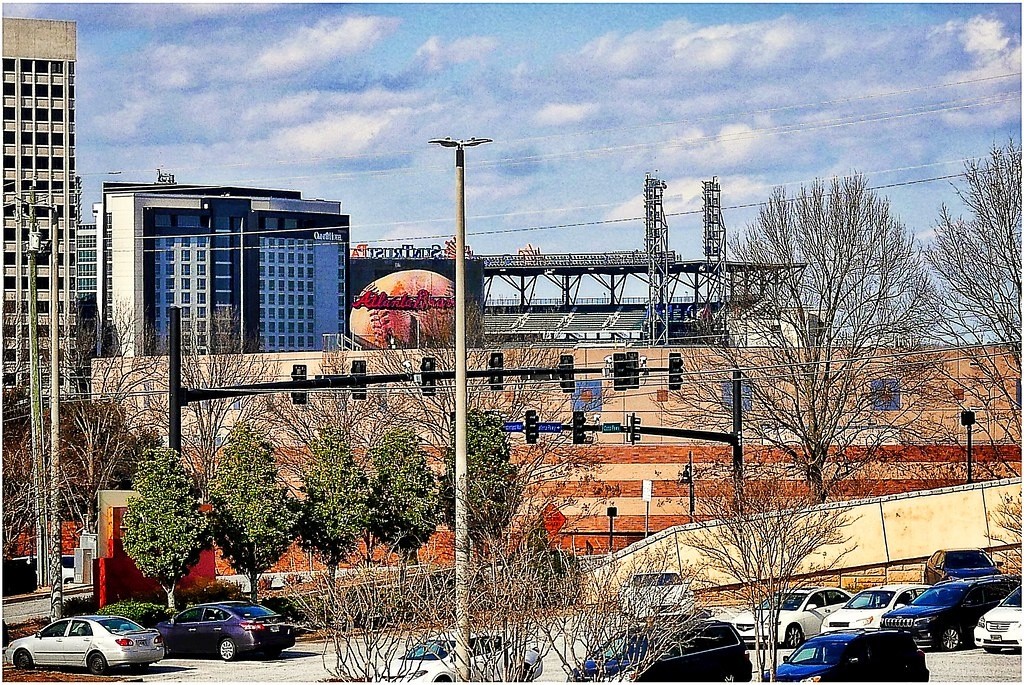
[961,410,975,485]
[680,465,695,524]
[428,136,491,682]
[606,506,617,553]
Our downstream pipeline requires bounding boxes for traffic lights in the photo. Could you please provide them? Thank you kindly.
[525,410,538,445]
[572,410,586,444]
[630,412,640,445]
[611,353,629,391]
[666,353,683,390]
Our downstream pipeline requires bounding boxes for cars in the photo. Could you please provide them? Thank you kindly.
[152,600,296,662]
[973,584,1022,653]
[15,555,73,585]
[620,569,693,621]
[733,586,930,650]
[924,548,1004,586]
[371,631,543,682]
[5,615,165,676]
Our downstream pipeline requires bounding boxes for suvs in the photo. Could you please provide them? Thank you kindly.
[763,629,930,682]
[567,619,752,683]
[880,575,1022,653]
